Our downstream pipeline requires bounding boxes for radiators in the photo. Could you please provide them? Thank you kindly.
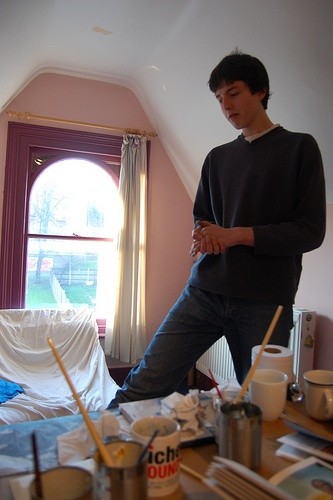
[195,307,317,391]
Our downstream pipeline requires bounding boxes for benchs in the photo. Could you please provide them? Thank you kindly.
[0,309,121,425]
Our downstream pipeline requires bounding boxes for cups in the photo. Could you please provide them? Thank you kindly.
[213,391,249,445]
[218,402,262,470]
[27,466,94,500]
[129,415,180,498]
[93,441,147,500]
[247,369,288,421]
[303,369,333,423]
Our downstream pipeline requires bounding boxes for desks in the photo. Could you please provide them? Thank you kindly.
[0,399,333,500]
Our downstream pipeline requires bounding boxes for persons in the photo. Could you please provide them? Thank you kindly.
[105,53,326,410]
